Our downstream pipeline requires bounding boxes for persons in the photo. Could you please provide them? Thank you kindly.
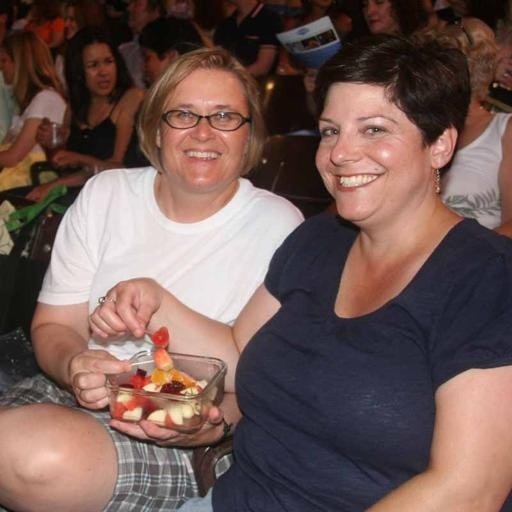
[85,27,512,512]
[411,16,510,236]
[3,2,205,236]
[360,2,429,39]
[418,2,510,113]
[0,45,308,512]
[189,2,371,123]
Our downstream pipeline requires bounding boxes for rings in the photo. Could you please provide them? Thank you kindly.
[97,295,117,305]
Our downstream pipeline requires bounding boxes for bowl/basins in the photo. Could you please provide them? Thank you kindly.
[105,349,228,435]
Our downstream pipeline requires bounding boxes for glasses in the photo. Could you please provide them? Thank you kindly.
[450,16,473,45]
[162,109,251,131]
